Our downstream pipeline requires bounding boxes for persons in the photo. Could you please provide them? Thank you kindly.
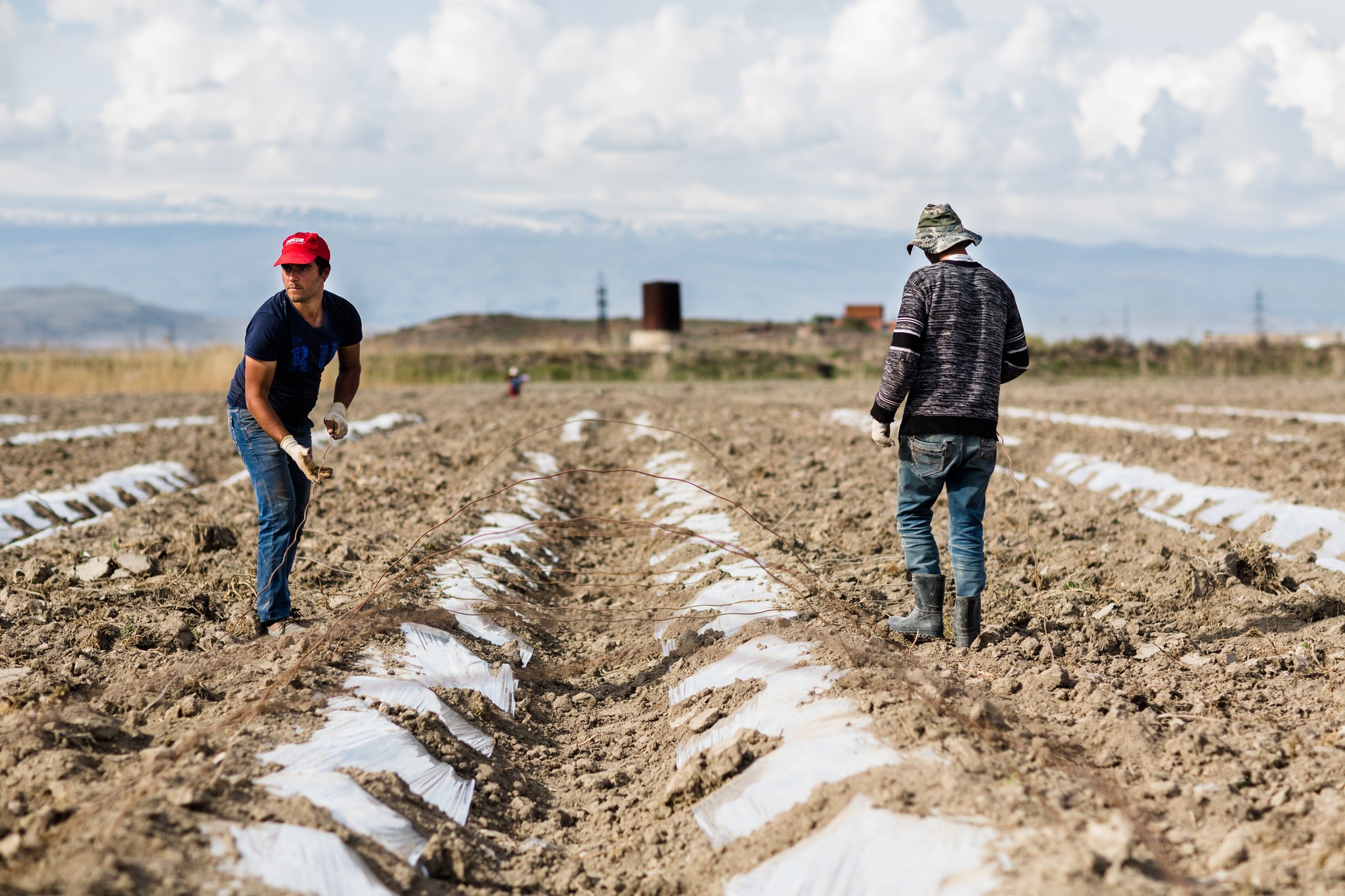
[866,200,1031,656]
[225,231,363,639]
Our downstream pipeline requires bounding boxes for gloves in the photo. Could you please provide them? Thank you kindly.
[323,402,349,440]
[872,419,895,448]
[280,435,333,485]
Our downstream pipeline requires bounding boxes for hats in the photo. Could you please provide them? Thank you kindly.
[273,232,331,267]
[906,202,982,256]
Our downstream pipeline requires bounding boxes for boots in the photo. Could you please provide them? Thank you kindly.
[888,572,946,637]
[953,593,981,649]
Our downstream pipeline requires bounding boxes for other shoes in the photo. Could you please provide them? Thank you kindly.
[267,607,307,638]
